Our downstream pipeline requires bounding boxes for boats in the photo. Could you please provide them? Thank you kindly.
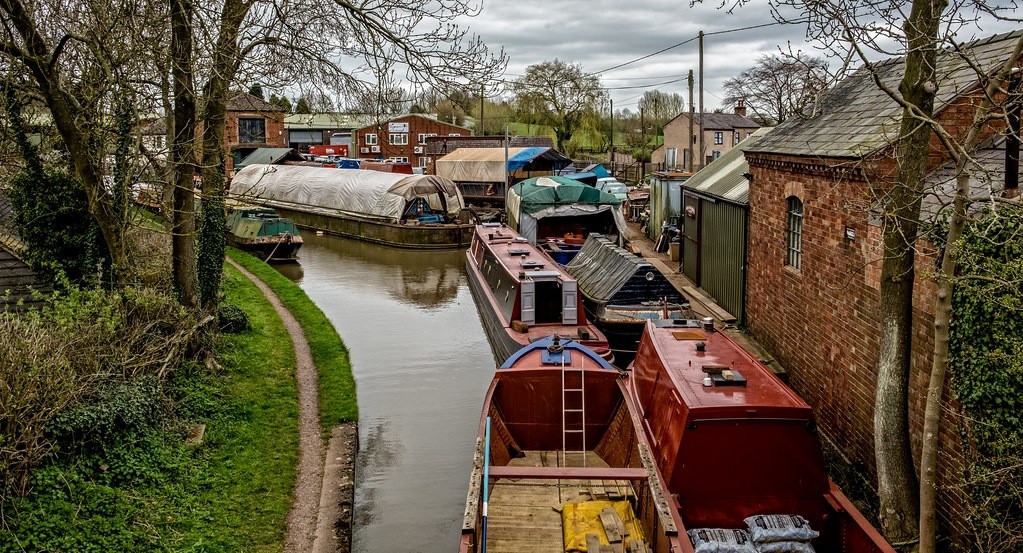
[436,145,580,205]
[227,164,501,248]
[464,223,617,367]
[623,316,894,553]
[504,175,699,341]
[459,332,682,553]
[133,188,304,264]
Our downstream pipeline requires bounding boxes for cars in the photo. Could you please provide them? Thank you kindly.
[598,182,629,202]
[596,177,620,189]
